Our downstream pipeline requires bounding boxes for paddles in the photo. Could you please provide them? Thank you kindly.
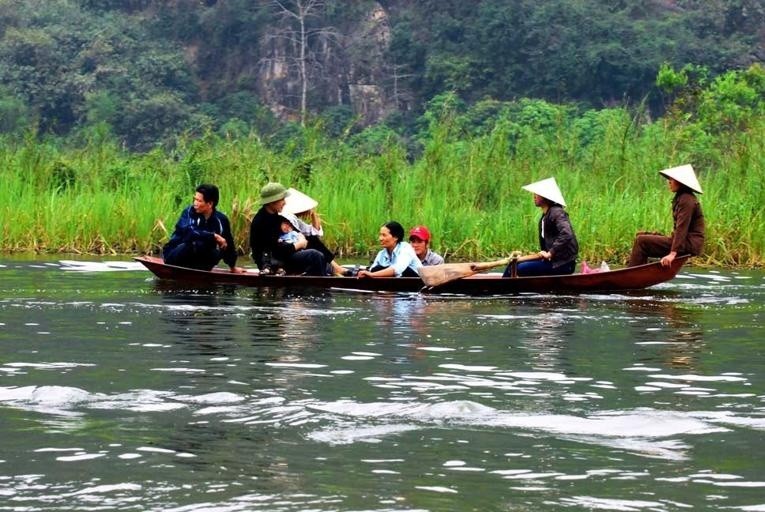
[418,254,545,286]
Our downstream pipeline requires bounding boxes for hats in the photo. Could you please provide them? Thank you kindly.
[259,181,291,205]
[658,163,703,194]
[408,225,429,240]
[520,177,567,206]
[278,187,319,214]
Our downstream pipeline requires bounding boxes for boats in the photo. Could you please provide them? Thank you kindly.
[136,254,691,292]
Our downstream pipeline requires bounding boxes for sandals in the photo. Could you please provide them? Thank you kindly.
[259,267,286,277]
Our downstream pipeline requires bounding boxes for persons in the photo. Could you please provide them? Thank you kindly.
[502,177,579,278]
[163,184,248,273]
[626,163,705,269]
[249,182,444,279]
